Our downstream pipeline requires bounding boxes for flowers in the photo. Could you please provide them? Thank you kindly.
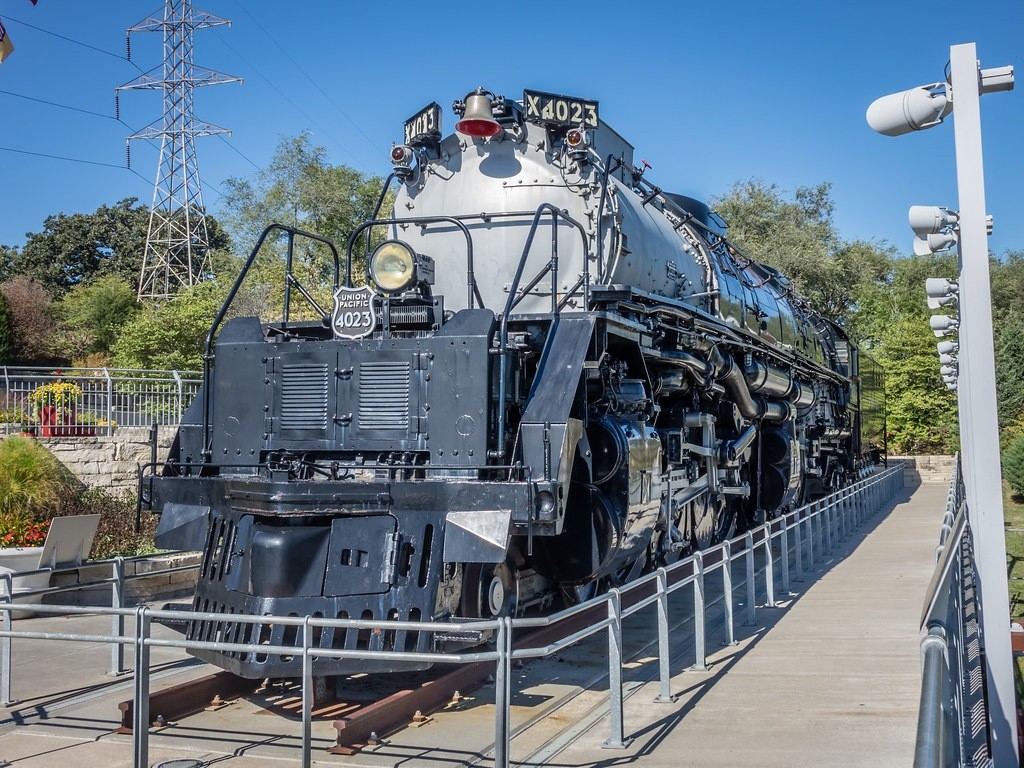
[20,413,39,433]
[28,382,83,425]
[95,416,117,431]
[76,411,98,425]
[0,513,53,551]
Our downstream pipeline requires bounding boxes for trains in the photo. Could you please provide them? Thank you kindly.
[136,87,864,702]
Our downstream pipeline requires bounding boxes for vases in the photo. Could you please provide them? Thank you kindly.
[19,431,37,437]
[36,403,76,436]
[0,547,52,622]
[95,426,115,436]
[77,422,96,436]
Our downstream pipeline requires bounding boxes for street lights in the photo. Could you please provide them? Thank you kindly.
[866,38,1022,768]
